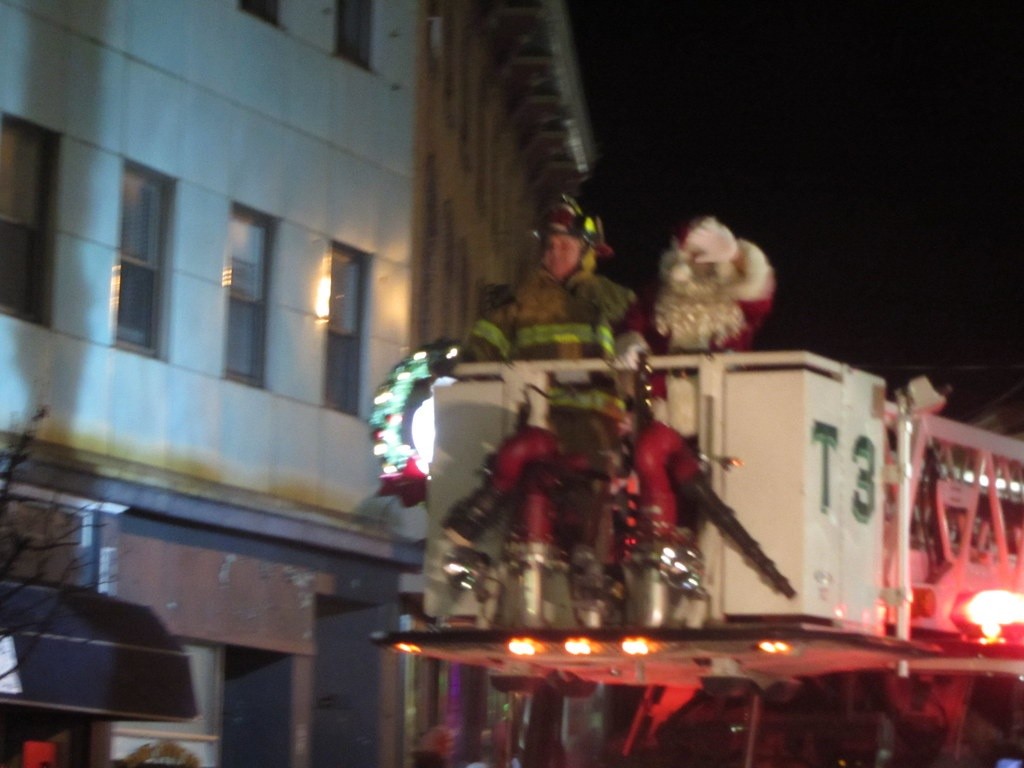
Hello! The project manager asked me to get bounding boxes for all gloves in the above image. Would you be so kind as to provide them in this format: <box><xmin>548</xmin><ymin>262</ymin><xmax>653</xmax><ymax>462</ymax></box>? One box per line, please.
<box><xmin>684</xmin><ymin>217</ymin><xmax>738</xmax><ymax>266</ymax></box>
<box><xmin>443</xmin><ymin>343</ymin><xmax>483</xmax><ymax>370</ymax></box>
<box><xmin>605</xmin><ymin>326</ymin><xmax>649</xmax><ymax>372</ymax></box>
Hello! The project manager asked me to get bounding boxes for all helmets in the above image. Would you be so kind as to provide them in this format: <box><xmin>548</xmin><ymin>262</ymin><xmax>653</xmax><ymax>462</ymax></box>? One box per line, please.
<box><xmin>526</xmin><ymin>194</ymin><xmax>613</xmax><ymax>257</ymax></box>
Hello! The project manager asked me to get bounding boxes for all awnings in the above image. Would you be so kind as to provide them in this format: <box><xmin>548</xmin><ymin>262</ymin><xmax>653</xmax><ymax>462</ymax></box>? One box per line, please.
<box><xmin>0</xmin><ymin>574</ymin><xmax>200</xmax><ymax>724</ymax></box>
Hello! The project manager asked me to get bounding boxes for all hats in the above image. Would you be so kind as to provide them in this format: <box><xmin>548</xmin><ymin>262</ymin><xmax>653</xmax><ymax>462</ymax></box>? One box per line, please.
<box><xmin>671</xmin><ymin>210</ymin><xmax>710</xmax><ymax>247</ymax></box>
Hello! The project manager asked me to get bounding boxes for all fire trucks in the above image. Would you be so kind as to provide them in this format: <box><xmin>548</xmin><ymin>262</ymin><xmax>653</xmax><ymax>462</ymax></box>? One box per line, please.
<box><xmin>371</xmin><ymin>349</ymin><xmax>1024</xmax><ymax>768</ymax></box>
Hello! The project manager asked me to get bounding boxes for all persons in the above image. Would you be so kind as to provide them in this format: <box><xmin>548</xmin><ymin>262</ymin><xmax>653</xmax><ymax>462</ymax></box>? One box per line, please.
<box><xmin>423</xmin><ymin>195</ymin><xmax>699</xmax><ymax>639</ymax></box>
<box><xmin>411</xmin><ymin>725</ymin><xmax>459</xmax><ymax>767</ymax></box>
